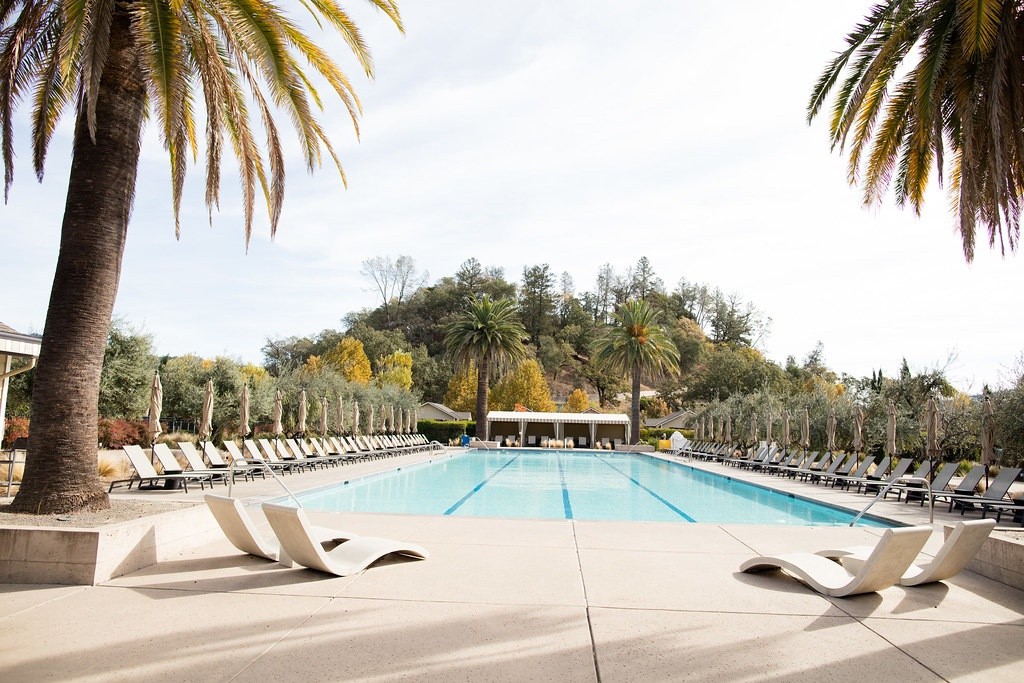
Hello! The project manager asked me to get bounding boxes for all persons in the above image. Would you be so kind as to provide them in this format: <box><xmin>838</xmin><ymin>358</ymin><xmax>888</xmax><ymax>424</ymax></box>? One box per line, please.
<box><xmin>661</xmin><ymin>433</ymin><xmax>668</xmax><ymax>439</ymax></box>
<box><xmin>725</xmin><ymin>415</ymin><xmax>732</xmax><ymax>453</ymax></box>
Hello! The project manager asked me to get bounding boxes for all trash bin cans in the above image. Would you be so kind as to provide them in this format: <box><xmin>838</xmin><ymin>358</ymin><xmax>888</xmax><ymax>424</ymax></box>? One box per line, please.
<box><xmin>462</xmin><ymin>435</ymin><xmax>471</xmax><ymax>447</ymax></box>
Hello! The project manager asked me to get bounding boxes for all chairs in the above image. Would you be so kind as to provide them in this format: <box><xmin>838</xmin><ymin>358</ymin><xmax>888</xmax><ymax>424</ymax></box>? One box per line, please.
<box><xmin>659</xmin><ymin>441</ymin><xmax>1024</xmax><ymax>528</ymax></box>
<box><xmin>108</xmin><ymin>431</ymin><xmax>440</xmax><ymax>492</ymax></box>
<box><xmin>204</xmin><ymin>495</ymin><xmax>357</xmax><ymax>562</ymax></box>
<box><xmin>813</xmin><ymin>519</ymin><xmax>997</xmax><ymax>586</ymax></box>
<box><xmin>495</xmin><ymin>436</ymin><xmax>621</xmax><ymax>449</ymax></box>
<box><xmin>739</xmin><ymin>527</ymin><xmax>933</xmax><ymax>597</ymax></box>
<box><xmin>261</xmin><ymin>503</ymin><xmax>427</xmax><ymax>576</ymax></box>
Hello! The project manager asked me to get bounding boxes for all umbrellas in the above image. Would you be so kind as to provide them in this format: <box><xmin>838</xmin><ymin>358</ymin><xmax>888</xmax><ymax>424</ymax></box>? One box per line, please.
<box><xmin>405</xmin><ymin>409</ymin><xmax>411</xmax><ymax>436</ymax></box>
<box><xmin>822</xmin><ymin>406</ymin><xmax>836</xmax><ymax>481</ymax></box>
<box><xmin>708</xmin><ymin>416</ymin><xmax>714</xmax><ymax>442</ymax></box>
<box><xmin>380</xmin><ymin>403</ymin><xmax>387</xmax><ymax>438</ymax></box>
<box><xmin>294</xmin><ymin>388</ymin><xmax>307</xmax><ymax>466</ymax></box>
<box><xmin>850</xmin><ymin>386</ymin><xmax>999</xmax><ymax>512</ymax></box>
<box><xmin>234</xmin><ymin>382</ymin><xmax>252</xmax><ymax>475</ymax></box>
<box><xmin>270</xmin><ymin>387</ymin><xmax>283</xmax><ymax>470</ymax></box>
<box><xmin>766</xmin><ymin>413</ymin><xmax>773</xmax><ymax>469</ymax></box>
<box><xmin>389</xmin><ymin>404</ymin><xmax>396</xmax><ymax>436</ymax></box>
<box><xmin>138</xmin><ymin>371</ymin><xmax>164</xmax><ymax>490</ymax></box>
<box><xmin>397</xmin><ymin>405</ymin><xmax>403</xmax><ymax>436</ymax></box>
<box><xmin>699</xmin><ymin>416</ymin><xmax>704</xmax><ymax>441</ymax></box>
<box><xmin>317</xmin><ymin>395</ymin><xmax>328</xmax><ymax>464</ymax></box>
<box><xmin>191</xmin><ymin>376</ymin><xmax>214</xmax><ymax>481</ymax></box>
<box><xmin>798</xmin><ymin>408</ymin><xmax>811</xmax><ymax>477</ymax></box>
<box><xmin>750</xmin><ymin>413</ymin><xmax>757</xmax><ymax>458</ymax></box>
<box><xmin>337</xmin><ymin>396</ymin><xmax>343</xmax><ymax>448</ymax></box>
<box><xmin>717</xmin><ymin>416</ymin><xmax>723</xmax><ymax>442</ymax></box>
<box><xmin>412</xmin><ymin>409</ymin><xmax>418</xmax><ymax>434</ymax></box>
<box><xmin>781</xmin><ymin>409</ymin><xmax>791</xmax><ymax>462</ymax></box>
<box><xmin>368</xmin><ymin>403</ymin><xmax>373</xmax><ymax>442</ymax></box>
<box><xmin>353</xmin><ymin>402</ymin><xmax>358</xmax><ymax>442</ymax></box>
<box><xmin>693</xmin><ymin>420</ymin><xmax>699</xmax><ymax>441</ymax></box>
